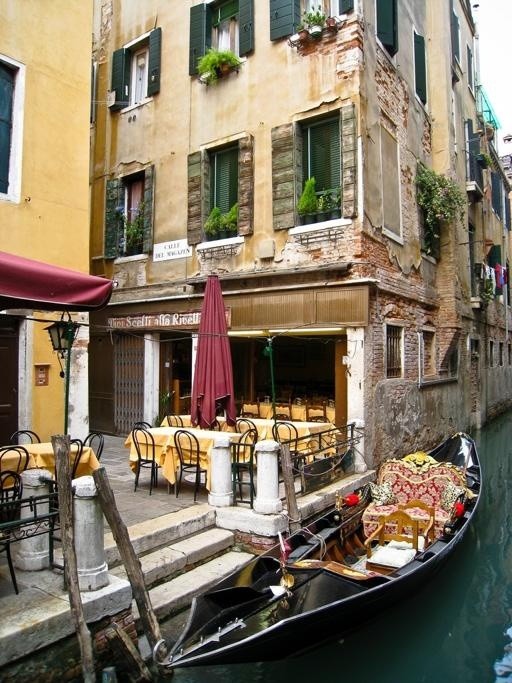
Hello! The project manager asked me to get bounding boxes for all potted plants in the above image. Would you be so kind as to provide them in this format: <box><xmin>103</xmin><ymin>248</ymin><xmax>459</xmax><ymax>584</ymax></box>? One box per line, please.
<box><xmin>204</xmin><ymin>202</ymin><xmax>237</xmax><ymax>241</ymax></box>
<box><xmin>294</xmin><ymin>6</ymin><xmax>336</xmax><ymax>41</ymax></box>
<box><xmin>196</xmin><ymin>46</ymin><xmax>241</xmax><ymax>87</ymax></box>
<box><xmin>296</xmin><ymin>176</ymin><xmax>341</xmax><ymax>225</ymax></box>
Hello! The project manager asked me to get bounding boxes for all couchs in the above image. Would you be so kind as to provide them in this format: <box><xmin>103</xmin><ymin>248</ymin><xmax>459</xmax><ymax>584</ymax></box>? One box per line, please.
<box><xmin>360</xmin><ymin>451</ymin><xmax>468</xmax><ymax>542</ymax></box>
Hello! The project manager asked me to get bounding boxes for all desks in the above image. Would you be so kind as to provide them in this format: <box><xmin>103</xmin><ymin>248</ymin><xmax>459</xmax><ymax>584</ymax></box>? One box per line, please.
<box><xmin>124</xmin><ymin>395</ymin><xmax>342</xmax><ymax>502</ymax></box>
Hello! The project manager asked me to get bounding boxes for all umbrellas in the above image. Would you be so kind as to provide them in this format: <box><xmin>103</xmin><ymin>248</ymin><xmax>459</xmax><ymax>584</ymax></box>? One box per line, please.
<box><xmin>185</xmin><ymin>272</ymin><xmax>239</xmax><ymax>432</ymax></box>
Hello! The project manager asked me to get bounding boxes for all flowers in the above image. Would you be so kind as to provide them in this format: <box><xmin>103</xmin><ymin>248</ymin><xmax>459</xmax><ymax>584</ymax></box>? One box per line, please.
<box><xmin>115</xmin><ymin>202</ymin><xmax>146</xmax><ymax>248</ymax></box>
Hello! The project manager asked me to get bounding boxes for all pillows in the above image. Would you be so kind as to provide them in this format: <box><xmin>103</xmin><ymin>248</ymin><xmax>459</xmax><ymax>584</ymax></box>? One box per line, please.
<box><xmin>436</xmin><ymin>480</ymin><xmax>467</xmax><ymax>515</ymax></box>
<box><xmin>369</xmin><ymin>480</ymin><xmax>399</xmax><ymax>506</ymax></box>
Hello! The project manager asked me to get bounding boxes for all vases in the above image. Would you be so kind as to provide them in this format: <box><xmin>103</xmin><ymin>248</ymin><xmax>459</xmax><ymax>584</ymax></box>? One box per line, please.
<box><xmin>127</xmin><ymin>248</ymin><xmax>141</xmax><ymax>255</ymax></box>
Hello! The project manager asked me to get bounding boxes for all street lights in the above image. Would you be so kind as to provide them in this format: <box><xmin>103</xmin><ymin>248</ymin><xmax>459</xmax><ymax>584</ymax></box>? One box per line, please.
<box><xmin>42</xmin><ymin>311</ymin><xmax>81</xmax><ymax>435</ymax></box>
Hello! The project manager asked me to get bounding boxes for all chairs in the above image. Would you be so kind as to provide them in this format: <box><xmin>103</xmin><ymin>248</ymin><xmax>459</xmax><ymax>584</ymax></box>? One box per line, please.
<box><xmin>364</xmin><ymin>510</ymin><xmax>418</xmax><ymax>575</ymax></box>
<box><xmin>386</xmin><ymin>499</ymin><xmax>435</xmax><ymax>553</ymax></box>
<box><xmin>0</xmin><ymin>430</ymin><xmax>104</xmax><ymax>594</ymax></box>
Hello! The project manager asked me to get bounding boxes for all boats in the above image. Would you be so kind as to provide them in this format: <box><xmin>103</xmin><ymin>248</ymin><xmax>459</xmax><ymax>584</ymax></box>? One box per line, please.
<box><xmin>152</xmin><ymin>431</ymin><xmax>483</xmax><ymax>670</ymax></box>
